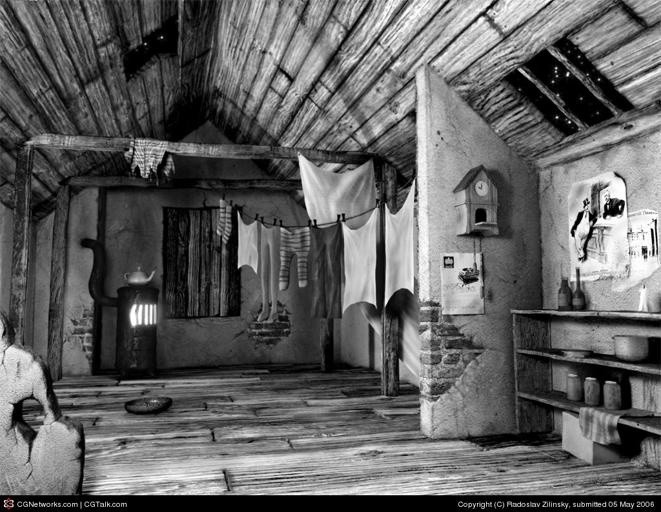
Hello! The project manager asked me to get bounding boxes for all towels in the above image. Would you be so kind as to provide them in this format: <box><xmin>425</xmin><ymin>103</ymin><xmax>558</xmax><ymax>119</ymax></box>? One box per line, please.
<box><xmin>331</xmin><ymin>179</ymin><xmax>418</xmax><ymax>313</ymax></box>
<box><xmin>236</xmin><ymin>211</ymin><xmax>259</xmax><ymax>274</ymax></box>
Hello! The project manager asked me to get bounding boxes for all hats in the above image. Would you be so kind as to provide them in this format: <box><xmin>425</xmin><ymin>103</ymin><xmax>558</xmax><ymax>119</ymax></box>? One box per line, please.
<box><xmin>582</xmin><ymin>198</ymin><xmax>590</xmax><ymax>208</ymax></box>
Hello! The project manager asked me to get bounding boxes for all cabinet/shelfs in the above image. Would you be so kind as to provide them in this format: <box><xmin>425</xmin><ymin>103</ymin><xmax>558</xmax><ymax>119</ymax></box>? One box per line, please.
<box><xmin>507</xmin><ymin>309</ymin><xmax>660</xmax><ymax>434</ymax></box>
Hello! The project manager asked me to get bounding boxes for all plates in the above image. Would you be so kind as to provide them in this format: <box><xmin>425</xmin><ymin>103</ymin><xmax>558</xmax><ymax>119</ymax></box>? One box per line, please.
<box><xmin>563</xmin><ymin>349</ymin><xmax>592</xmax><ymax>358</ymax></box>
<box><xmin>125</xmin><ymin>397</ymin><xmax>171</xmax><ymax>414</ymax></box>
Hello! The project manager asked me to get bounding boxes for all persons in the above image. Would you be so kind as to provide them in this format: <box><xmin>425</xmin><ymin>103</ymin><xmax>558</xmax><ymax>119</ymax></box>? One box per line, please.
<box><xmin>602</xmin><ymin>191</ymin><xmax>625</xmax><ymax>220</ymax></box>
<box><xmin>571</xmin><ymin>199</ymin><xmax>597</xmax><ymax>262</ymax></box>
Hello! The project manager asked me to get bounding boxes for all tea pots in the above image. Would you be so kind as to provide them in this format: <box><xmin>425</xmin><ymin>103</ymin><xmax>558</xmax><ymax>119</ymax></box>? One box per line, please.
<box><xmin>123</xmin><ymin>266</ymin><xmax>155</xmax><ymax>286</ymax></box>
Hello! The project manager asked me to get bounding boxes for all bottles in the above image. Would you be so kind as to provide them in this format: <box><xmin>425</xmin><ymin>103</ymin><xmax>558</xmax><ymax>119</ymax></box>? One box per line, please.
<box><xmin>564</xmin><ymin>374</ymin><xmax>622</xmax><ymax>411</ymax></box>
<box><xmin>571</xmin><ymin>267</ymin><xmax>586</xmax><ymax>310</ymax></box>
<box><xmin>557</xmin><ymin>277</ymin><xmax>573</xmax><ymax>311</ymax></box>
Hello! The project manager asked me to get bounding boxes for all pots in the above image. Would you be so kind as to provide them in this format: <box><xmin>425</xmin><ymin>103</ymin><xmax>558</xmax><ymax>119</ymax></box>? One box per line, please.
<box><xmin>613</xmin><ymin>336</ymin><xmax>649</xmax><ymax>361</ymax></box>
